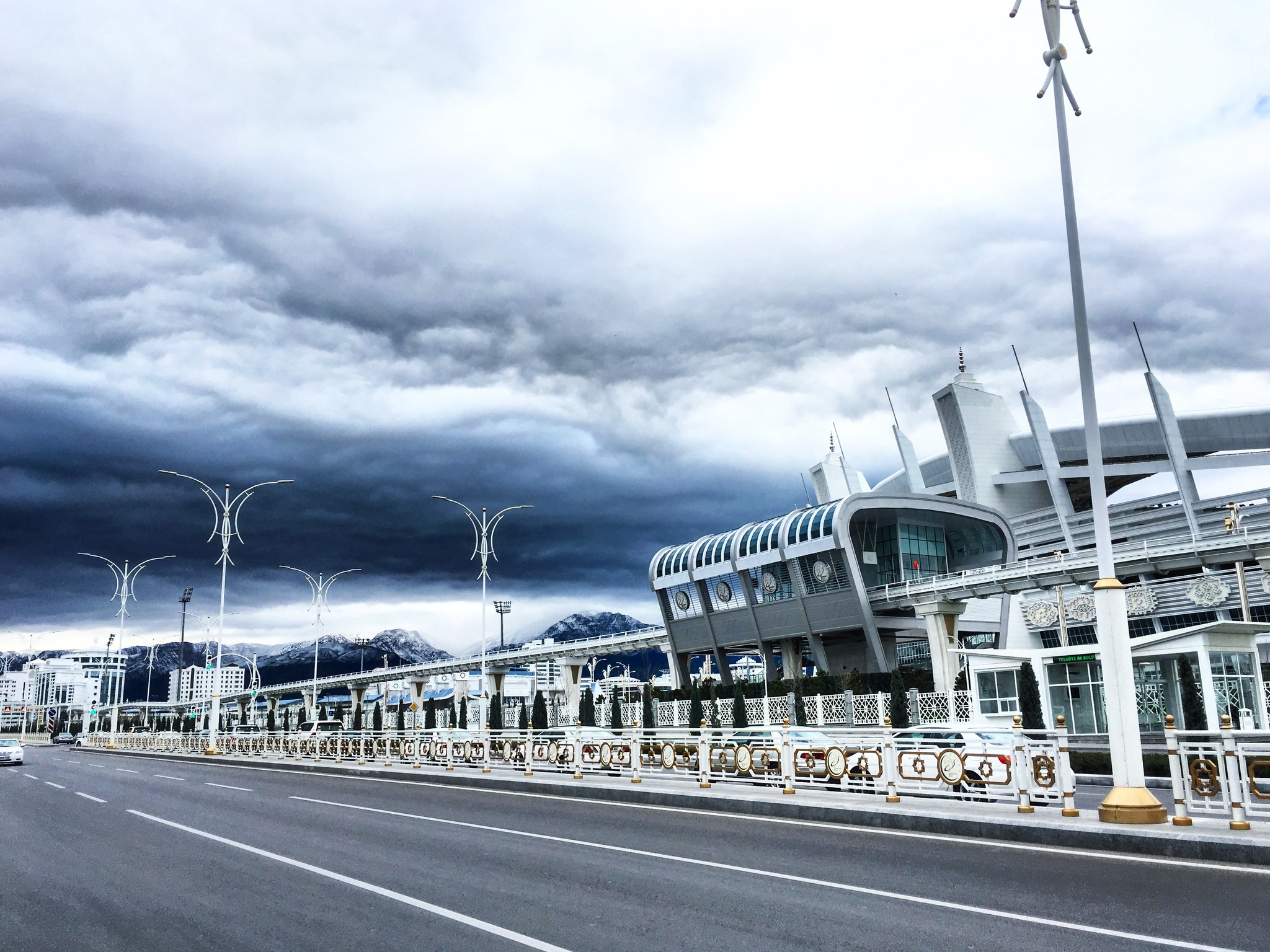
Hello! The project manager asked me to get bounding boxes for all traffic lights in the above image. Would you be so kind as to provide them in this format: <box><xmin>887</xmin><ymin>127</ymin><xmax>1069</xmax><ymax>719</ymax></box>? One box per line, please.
<box><xmin>90</xmin><ymin>699</ymin><xmax>97</xmax><ymax>715</ymax></box>
<box><xmin>252</xmin><ymin>681</ymin><xmax>257</xmax><ymax>696</ymax></box>
<box><xmin>190</xmin><ymin>705</ymin><xmax>195</xmax><ymax>718</ymax></box>
<box><xmin>207</xmin><ymin>655</ymin><xmax>212</xmax><ymax>669</ymax></box>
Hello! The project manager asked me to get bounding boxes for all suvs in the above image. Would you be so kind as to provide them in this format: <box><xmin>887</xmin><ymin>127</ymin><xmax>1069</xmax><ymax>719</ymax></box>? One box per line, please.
<box><xmin>219</xmin><ymin>724</ymin><xmax>262</xmax><ymax>744</ymax></box>
<box><xmin>125</xmin><ymin>726</ymin><xmax>152</xmax><ymax>739</ymax></box>
<box><xmin>287</xmin><ymin>719</ymin><xmax>346</xmax><ymax>751</ymax></box>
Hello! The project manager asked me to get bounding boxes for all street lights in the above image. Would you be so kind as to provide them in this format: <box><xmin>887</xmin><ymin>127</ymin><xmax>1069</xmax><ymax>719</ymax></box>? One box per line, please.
<box><xmin>2</xmin><ymin>630</ymin><xmax>61</xmax><ymax>740</ymax></box>
<box><xmin>433</xmin><ymin>495</ymin><xmax>536</xmax><ymax>741</ymax></box>
<box><xmin>76</xmin><ymin>552</ymin><xmax>178</xmax><ymax>751</ymax></box>
<box><xmin>101</xmin><ymin>633</ymin><xmax>115</xmax><ymax>706</ymax></box>
<box><xmin>178</xmin><ymin>611</ymin><xmax>240</xmax><ymax>753</ymax></box>
<box><xmin>176</xmin><ymin>586</ymin><xmax>194</xmax><ymax>701</ymax></box>
<box><xmin>129</xmin><ymin>633</ymin><xmax>180</xmax><ymax>727</ymax></box>
<box><xmin>381</xmin><ymin>656</ymin><xmax>389</xmax><ymax>713</ymax></box>
<box><xmin>493</xmin><ymin>601</ymin><xmax>512</xmax><ymax>648</ymax></box>
<box><xmin>158</xmin><ymin>468</ymin><xmax>296</xmax><ymax>753</ymax></box>
<box><xmin>270</xmin><ymin>697</ymin><xmax>279</xmax><ymax>727</ymax></box>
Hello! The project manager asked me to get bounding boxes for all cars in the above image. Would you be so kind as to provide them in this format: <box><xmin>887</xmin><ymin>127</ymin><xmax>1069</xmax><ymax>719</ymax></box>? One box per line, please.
<box><xmin>75</xmin><ymin>732</ymin><xmax>111</xmax><ymax>747</ymax></box>
<box><xmin>73</xmin><ymin>732</ymin><xmax>87</xmax><ymax>742</ymax></box>
<box><xmin>0</xmin><ymin>738</ymin><xmax>26</xmax><ymax>766</ymax></box>
<box><xmin>695</xmin><ymin>724</ymin><xmax>840</xmax><ymax>788</ymax></box>
<box><xmin>845</xmin><ymin>725</ymin><xmax>1077</xmax><ymax>806</ymax></box>
<box><xmin>186</xmin><ymin>729</ymin><xmax>221</xmax><ymax>737</ymax></box>
<box><xmin>325</xmin><ymin>730</ymin><xmax>376</xmax><ymax>762</ymax></box>
<box><xmin>400</xmin><ymin>727</ymin><xmax>480</xmax><ymax>768</ymax></box>
<box><xmin>157</xmin><ymin>731</ymin><xmax>185</xmax><ymax>739</ymax></box>
<box><xmin>510</xmin><ymin>724</ymin><xmax>623</xmax><ymax>776</ymax></box>
<box><xmin>53</xmin><ymin>732</ymin><xmax>73</xmax><ymax>745</ymax></box>
